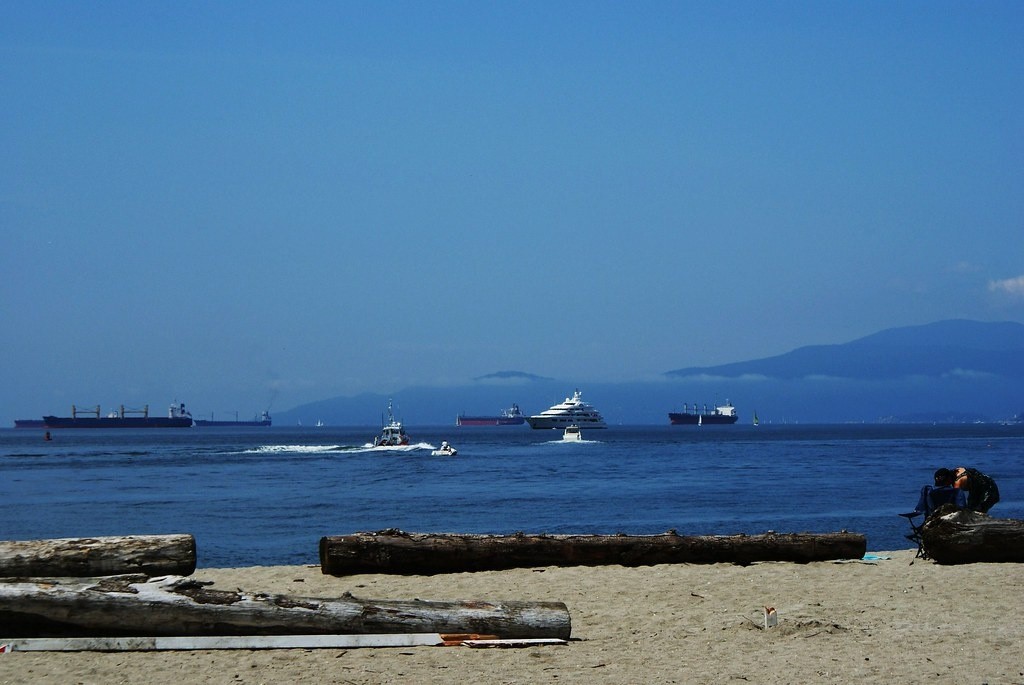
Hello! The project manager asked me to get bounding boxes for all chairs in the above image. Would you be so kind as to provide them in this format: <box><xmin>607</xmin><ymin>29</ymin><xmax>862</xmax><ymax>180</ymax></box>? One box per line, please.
<box><xmin>898</xmin><ymin>481</ymin><xmax>965</xmax><ymax>566</ymax></box>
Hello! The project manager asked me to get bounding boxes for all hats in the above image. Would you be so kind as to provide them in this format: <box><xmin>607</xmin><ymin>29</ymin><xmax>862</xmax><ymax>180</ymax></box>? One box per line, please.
<box><xmin>934</xmin><ymin>468</ymin><xmax>950</xmax><ymax>486</ymax></box>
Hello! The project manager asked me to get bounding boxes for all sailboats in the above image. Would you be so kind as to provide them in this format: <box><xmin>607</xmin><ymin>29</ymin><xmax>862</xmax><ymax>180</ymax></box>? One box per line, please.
<box><xmin>296</xmin><ymin>416</ymin><xmax>304</xmax><ymax>427</ymax></box>
<box><xmin>315</xmin><ymin>419</ymin><xmax>324</xmax><ymax>427</ymax></box>
<box><xmin>752</xmin><ymin>410</ymin><xmax>760</xmax><ymax>432</ymax></box>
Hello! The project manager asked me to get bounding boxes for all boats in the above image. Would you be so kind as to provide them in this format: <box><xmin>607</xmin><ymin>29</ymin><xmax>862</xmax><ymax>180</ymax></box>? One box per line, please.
<box><xmin>562</xmin><ymin>424</ymin><xmax>582</xmax><ymax>441</ymax></box>
<box><xmin>195</xmin><ymin>419</ymin><xmax>272</xmax><ymax>427</ymax></box>
<box><xmin>374</xmin><ymin>422</ymin><xmax>411</xmax><ymax>446</ymax></box>
<box><xmin>456</xmin><ymin>403</ymin><xmax>525</xmax><ymax>426</ymax></box>
<box><xmin>431</xmin><ymin>445</ymin><xmax>457</xmax><ymax>457</ymax></box>
<box><xmin>42</xmin><ymin>404</ymin><xmax>194</xmax><ymax>428</ymax></box>
<box><xmin>14</xmin><ymin>419</ymin><xmax>47</xmax><ymax>428</ymax></box>
<box><xmin>666</xmin><ymin>391</ymin><xmax>739</xmax><ymax>424</ymax></box>
<box><xmin>525</xmin><ymin>388</ymin><xmax>609</xmax><ymax>428</ymax></box>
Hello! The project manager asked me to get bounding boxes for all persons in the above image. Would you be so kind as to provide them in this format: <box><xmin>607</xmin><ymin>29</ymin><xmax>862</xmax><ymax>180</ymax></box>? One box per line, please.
<box><xmin>935</xmin><ymin>467</ymin><xmax>999</xmax><ymax>515</ymax></box>
<box><xmin>440</xmin><ymin>439</ymin><xmax>451</xmax><ymax>452</ymax></box>
